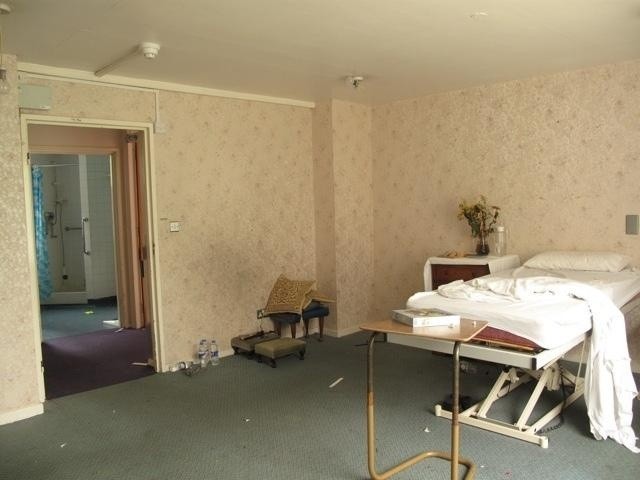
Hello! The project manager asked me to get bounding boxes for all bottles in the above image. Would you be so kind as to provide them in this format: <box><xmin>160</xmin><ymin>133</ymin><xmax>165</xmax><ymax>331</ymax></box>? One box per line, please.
<box><xmin>169</xmin><ymin>360</ymin><xmax>195</xmax><ymax>372</ymax></box>
<box><xmin>200</xmin><ymin>339</ymin><xmax>219</xmax><ymax>368</ymax></box>
<box><xmin>495</xmin><ymin>226</ymin><xmax>507</xmax><ymax>256</ymax></box>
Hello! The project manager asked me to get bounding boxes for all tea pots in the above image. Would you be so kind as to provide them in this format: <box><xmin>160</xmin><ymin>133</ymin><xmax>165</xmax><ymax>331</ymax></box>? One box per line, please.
<box><xmin>473</xmin><ymin>236</ymin><xmax>490</xmax><ymax>255</ymax></box>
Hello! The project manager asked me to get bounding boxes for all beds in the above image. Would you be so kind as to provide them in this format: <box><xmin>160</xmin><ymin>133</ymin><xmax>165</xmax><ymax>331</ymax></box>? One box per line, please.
<box><xmin>388</xmin><ymin>252</ymin><xmax>640</xmax><ymax>448</ymax></box>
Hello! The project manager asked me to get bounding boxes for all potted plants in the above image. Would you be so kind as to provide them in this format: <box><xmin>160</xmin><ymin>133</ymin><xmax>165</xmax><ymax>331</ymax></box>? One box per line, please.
<box><xmin>457</xmin><ymin>195</ymin><xmax>500</xmax><ymax>255</ymax></box>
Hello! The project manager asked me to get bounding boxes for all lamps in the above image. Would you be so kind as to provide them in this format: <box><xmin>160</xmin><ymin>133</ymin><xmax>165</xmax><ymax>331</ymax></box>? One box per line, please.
<box><xmin>0</xmin><ymin>67</ymin><xmax>11</xmax><ymax>95</ymax></box>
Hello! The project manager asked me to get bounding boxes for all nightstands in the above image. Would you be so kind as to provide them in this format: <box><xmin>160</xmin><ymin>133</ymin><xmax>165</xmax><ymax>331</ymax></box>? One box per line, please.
<box><xmin>424</xmin><ymin>255</ymin><xmax>520</xmax><ymax>290</ymax></box>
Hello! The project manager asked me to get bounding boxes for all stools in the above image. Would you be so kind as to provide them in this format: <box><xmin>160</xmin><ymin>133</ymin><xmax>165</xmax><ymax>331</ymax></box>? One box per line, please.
<box><xmin>271</xmin><ymin>306</ymin><xmax>329</xmax><ymax>342</ymax></box>
<box><xmin>254</xmin><ymin>337</ymin><xmax>307</xmax><ymax>368</ymax></box>
<box><xmin>231</xmin><ymin>332</ymin><xmax>280</xmax><ymax>360</ymax></box>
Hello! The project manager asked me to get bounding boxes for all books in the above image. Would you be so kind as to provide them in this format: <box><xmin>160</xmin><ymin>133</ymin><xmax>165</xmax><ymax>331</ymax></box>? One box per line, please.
<box><xmin>392</xmin><ymin>308</ymin><xmax>460</xmax><ymax>328</ymax></box>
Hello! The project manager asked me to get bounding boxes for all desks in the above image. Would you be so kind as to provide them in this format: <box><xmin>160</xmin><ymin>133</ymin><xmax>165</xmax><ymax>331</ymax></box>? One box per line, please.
<box><xmin>358</xmin><ymin>318</ymin><xmax>488</xmax><ymax>480</ymax></box>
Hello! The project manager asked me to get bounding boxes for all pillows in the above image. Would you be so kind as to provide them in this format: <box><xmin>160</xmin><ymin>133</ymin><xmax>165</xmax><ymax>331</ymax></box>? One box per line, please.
<box><xmin>263</xmin><ymin>273</ymin><xmax>316</xmax><ymax>317</ymax></box>
<box><xmin>303</xmin><ymin>290</ymin><xmax>337</xmax><ymax>311</ymax></box>
<box><xmin>521</xmin><ymin>252</ymin><xmax>631</xmax><ymax>272</ymax></box>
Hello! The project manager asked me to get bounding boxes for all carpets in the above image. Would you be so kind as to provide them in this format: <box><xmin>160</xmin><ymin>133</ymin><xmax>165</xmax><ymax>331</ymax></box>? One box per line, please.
<box><xmin>42</xmin><ymin>328</ymin><xmax>154</xmax><ymax>401</ymax></box>
